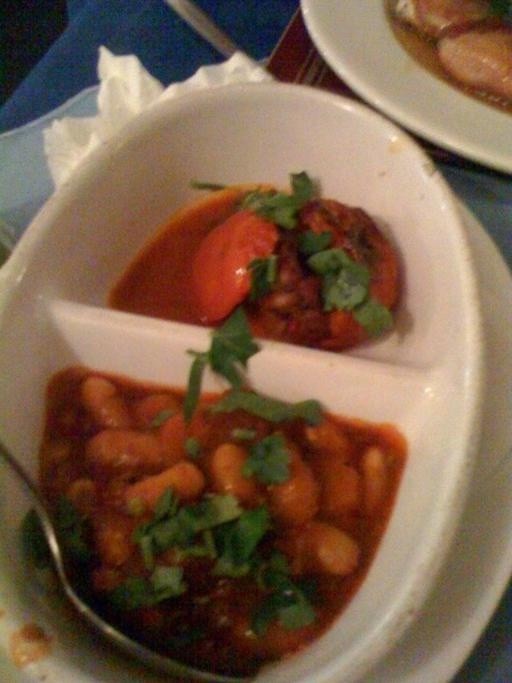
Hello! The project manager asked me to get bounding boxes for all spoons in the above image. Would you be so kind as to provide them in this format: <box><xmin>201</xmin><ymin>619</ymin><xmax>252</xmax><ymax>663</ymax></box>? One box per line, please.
<box><xmin>3</xmin><ymin>442</ymin><xmax>250</xmax><ymax>678</ymax></box>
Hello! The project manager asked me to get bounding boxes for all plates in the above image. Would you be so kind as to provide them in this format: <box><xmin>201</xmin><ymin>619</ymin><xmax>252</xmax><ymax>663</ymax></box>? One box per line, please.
<box><xmin>362</xmin><ymin>173</ymin><xmax>512</xmax><ymax>681</ymax></box>
<box><xmin>2</xmin><ymin>75</ymin><xmax>485</xmax><ymax>683</ymax></box>
<box><xmin>294</xmin><ymin>0</ymin><xmax>512</xmax><ymax>176</ymax></box>
